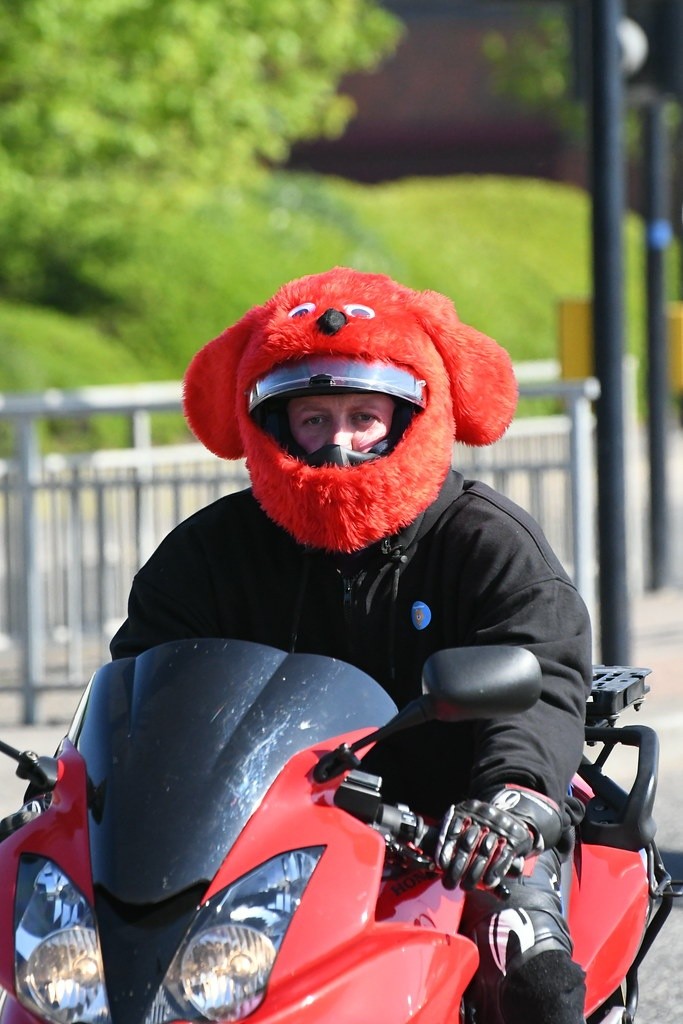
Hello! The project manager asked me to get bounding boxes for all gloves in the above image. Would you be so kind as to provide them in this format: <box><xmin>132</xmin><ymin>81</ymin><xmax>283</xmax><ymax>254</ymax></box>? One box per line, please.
<box><xmin>433</xmin><ymin>790</ymin><xmax>566</xmax><ymax>892</ymax></box>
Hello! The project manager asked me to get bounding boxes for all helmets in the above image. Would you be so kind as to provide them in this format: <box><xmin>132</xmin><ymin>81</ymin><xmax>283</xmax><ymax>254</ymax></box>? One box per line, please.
<box><xmin>248</xmin><ymin>353</ymin><xmax>427</xmax><ymax>467</ymax></box>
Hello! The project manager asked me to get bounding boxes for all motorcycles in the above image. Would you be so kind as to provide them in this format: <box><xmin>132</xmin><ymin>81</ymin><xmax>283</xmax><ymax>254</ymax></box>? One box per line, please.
<box><xmin>0</xmin><ymin>643</ymin><xmax>683</xmax><ymax>1024</ymax></box>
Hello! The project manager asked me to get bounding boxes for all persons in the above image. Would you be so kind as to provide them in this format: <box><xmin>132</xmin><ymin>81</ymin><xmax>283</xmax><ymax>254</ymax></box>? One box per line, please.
<box><xmin>105</xmin><ymin>262</ymin><xmax>593</xmax><ymax>1024</ymax></box>
